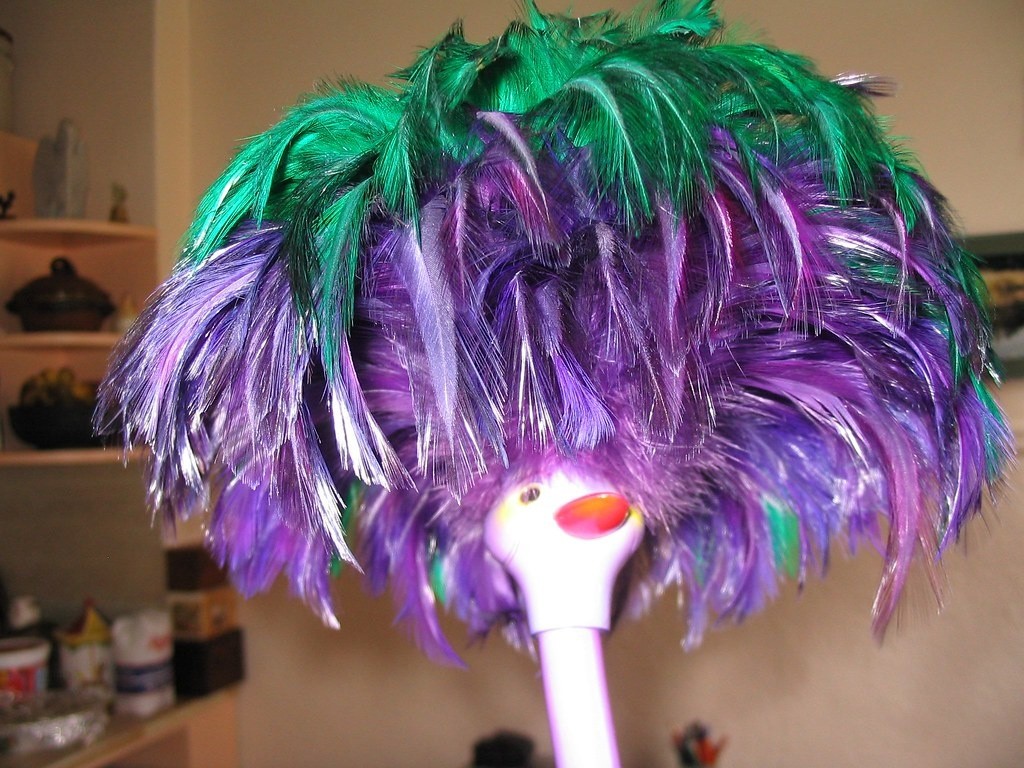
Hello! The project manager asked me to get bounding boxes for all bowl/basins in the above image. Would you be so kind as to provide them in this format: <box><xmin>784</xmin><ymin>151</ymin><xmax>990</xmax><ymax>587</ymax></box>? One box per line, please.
<box><xmin>9</xmin><ymin>405</ymin><xmax>138</xmax><ymax>448</ymax></box>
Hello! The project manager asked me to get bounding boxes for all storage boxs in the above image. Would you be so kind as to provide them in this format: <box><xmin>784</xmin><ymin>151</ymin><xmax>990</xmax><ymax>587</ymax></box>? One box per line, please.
<box><xmin>165</xmin><ymin>540</ymin><xmax>245</xmax><ymax>702</ymax></box>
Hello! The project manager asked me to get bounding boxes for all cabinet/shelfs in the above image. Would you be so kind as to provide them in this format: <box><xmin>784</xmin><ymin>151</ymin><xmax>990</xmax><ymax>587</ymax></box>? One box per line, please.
<box><xmin>0</xmin><ymin>129</ymin><xmax>159</xmax><ymax>463</ymax></box>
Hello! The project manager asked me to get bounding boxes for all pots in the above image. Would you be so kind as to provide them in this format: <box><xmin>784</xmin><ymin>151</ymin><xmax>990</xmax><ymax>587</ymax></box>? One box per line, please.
<box><xmin>4</xmin><ymin>258</ymin><xmax>116</xmax><ymax>331</ymax></box>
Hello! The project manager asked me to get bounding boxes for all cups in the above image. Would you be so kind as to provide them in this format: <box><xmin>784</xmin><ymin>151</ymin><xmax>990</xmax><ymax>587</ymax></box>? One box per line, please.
<box><xmin>0</xmin><ymin>638</ymin><xmax>49</xmax><ymax>717</ymax></box>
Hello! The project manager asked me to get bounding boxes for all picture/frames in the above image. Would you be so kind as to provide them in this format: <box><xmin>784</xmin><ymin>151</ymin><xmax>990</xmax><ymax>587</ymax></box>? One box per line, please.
<box><xmin>960</xmin><ymin>233</ymin><xmax>1023</xmax><ymax>381</ymax></box>
